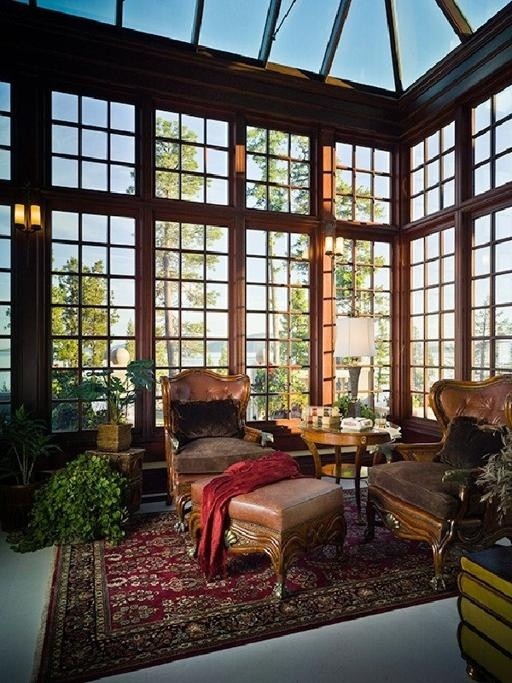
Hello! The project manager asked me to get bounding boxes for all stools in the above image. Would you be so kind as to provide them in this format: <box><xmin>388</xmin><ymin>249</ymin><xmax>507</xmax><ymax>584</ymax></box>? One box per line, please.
<box><xmin>187</xmin><ymin>468</ymin><xmax>349</xmax><ymax>603</ymax></box>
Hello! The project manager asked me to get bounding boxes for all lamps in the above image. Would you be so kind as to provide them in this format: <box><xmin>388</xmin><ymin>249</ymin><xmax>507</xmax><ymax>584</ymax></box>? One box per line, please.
<box><xmin>321</xmin><ymin>315</ymin><xmax>377</xmax><ymax>478</ymax></box>
<box><xmin>12</xmin><ymin>203</ymin><xmax>43</xmax><ymax>241</ymax></box>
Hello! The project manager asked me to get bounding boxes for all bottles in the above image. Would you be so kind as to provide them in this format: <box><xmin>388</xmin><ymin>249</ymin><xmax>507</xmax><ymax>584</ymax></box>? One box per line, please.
<box><xmin>345</xmin><ymin>391</ymin><xmax>351</xmax><ymax>412</ymax></box>
<box><xmin>311</xmin><ymin>407</ymin><xmax>329</xmax><ymax>429</ymax></box>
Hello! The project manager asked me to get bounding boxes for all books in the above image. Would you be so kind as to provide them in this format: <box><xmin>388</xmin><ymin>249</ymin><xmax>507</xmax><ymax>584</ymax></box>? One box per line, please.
<box><xmin>456</xmin><ymin>547</ymin><xmax>511</xmax><ymax>683</ymax></box>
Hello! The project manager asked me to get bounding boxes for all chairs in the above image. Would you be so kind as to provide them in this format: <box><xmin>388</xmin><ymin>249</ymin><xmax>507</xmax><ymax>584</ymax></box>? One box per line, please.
<box><xmin>364</xmin><ymin>373</ymin><xmax>512</xmax><ymax>591</ymax></box>
<box><xmin>160</xmin><ymin>369</ymin><xmax>278</xmax><ymax>531</ymax></box>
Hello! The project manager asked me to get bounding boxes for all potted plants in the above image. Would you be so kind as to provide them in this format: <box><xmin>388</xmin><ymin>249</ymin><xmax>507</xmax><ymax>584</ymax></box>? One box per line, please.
<box><xmin>0</xmin><ymin>403</ymin><xmax>59</xmax><ymax>522</ymax></box>
<box><xmin>72</xmin><ymin>359</ymin><xmax>155</xmax><ymax>454</ymax></box>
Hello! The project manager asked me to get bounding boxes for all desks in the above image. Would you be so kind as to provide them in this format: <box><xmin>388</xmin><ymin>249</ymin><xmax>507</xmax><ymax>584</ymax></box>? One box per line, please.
<box><xmin>86</xmin><ymin>447</ymin><xmax>145</xmax><ymax>514</ymax></box>
<box><xmin>301</xmin><ymin>425</ymin><xmax>392</xmax><ymax>506</ymax></box>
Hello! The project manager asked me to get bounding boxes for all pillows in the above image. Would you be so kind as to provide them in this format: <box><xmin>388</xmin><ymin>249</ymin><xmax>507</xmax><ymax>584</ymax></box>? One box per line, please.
<box><xmin>440</xmin><ymin>418</ymin><xmax>508</xmax><ymax>471</ymax></box>
<box><xmin>170</xmin><ymin>399</ymin><xmax>245</xmax><ymax>449</ymax></box>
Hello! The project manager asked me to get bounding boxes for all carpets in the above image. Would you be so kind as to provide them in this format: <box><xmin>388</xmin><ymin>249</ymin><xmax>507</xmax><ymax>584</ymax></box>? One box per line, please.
<box><xmin>20</xmin><ymin>485</ymin><xmax>463</xmax><ymax>682</ymax></box>
